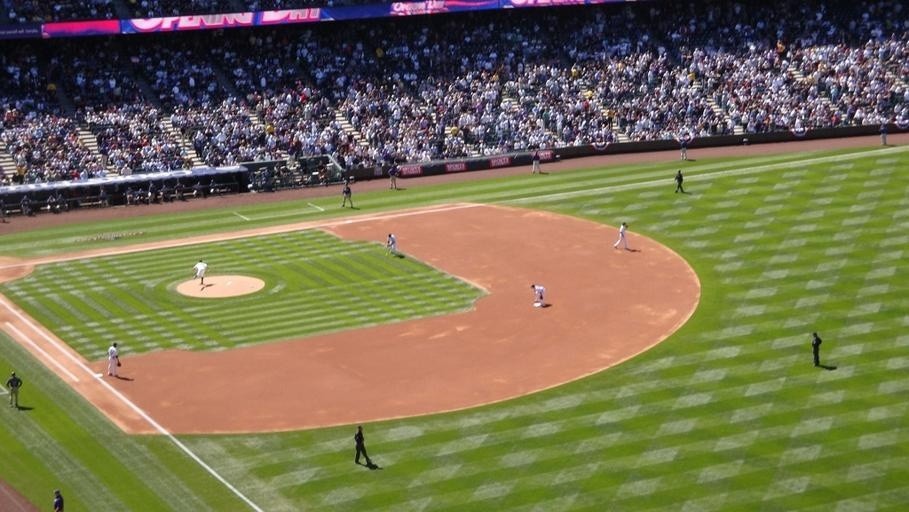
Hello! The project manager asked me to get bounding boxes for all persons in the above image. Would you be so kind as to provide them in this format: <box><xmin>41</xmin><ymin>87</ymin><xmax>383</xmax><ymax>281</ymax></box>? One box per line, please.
<box><xmin>6</xmin><ymin>370</ymin><xmax>23</xmax><ymax>407</ymax></box>
<box><xmin>2</xmin><ymin>1</ymin><xmax>909</xmax><ymax>198</ymax></box>
<box><xmin>108</xmin><ymin>343</ymin><xmax>120</xmax><ymax>376</ymax></box>
<box><xmin>354</xmin><ymin>425</ymin><xmax>372</xmax><ymax>465</ymax></box>
<box><xmin>19</xmin><ymin>195</ymin><xmax>32</xmax><ymax>216</ymax></box>
<box><xmin>193</xmin><ymin>260</ymin><xmax>208</xmax><ymax>283</ymax></box>
<box><xmin>56</xmin><ymin>194</ymin><xmax>66</xmax><ymax>210</ymax></box>
<box><xmin>47</xmin><ymin>195</ymin><xmax>56</xmax><ymax>208</ymax></box>
<box><xmin>614</xmin><ymin>222</ymin><xmax>628</xmax><ymax>248</ymax></box>
<box><xmin>530</xmin><ymin>285</ymin><xmax>546</xmax><ymax>306</ymax></box>
<box><xmin>99</xmin><ymin>189</ymin><xmax>108</xmax><ymax>208</ymax></box>
<box><xmin>126</xmin><ymin>185</ymin><xmax>133</xmax><ymax>205</ymax></box>
<box><xmin>54</xmin><ymin>490</ymin><xmax>64</xmax><ymax>511</ymax></box>
<box><xmin>811</xmin><ymin>333</ymin><xmax>823</xmax><ymax>366</ymax></box>
<box><xmin>341</xmin><ymin>182</ymin><xmax>354</xmax><ymax>208</ymax></box>
<box><xmin>387</xmin><ymin>233</ymin><xmax>398</xmax><ymax>256</ymax></box>
<box><xmin>0</xmin><ymin>198</ymin><xmax>8</xmax><ymax>223</ymax></box>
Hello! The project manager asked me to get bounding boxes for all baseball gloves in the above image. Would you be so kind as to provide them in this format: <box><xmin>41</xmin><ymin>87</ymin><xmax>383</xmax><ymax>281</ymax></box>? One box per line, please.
<box><xmin>117</xmin><ymin>363</ymin><xmax>122</xmax><ymax>367</ymax></box>
